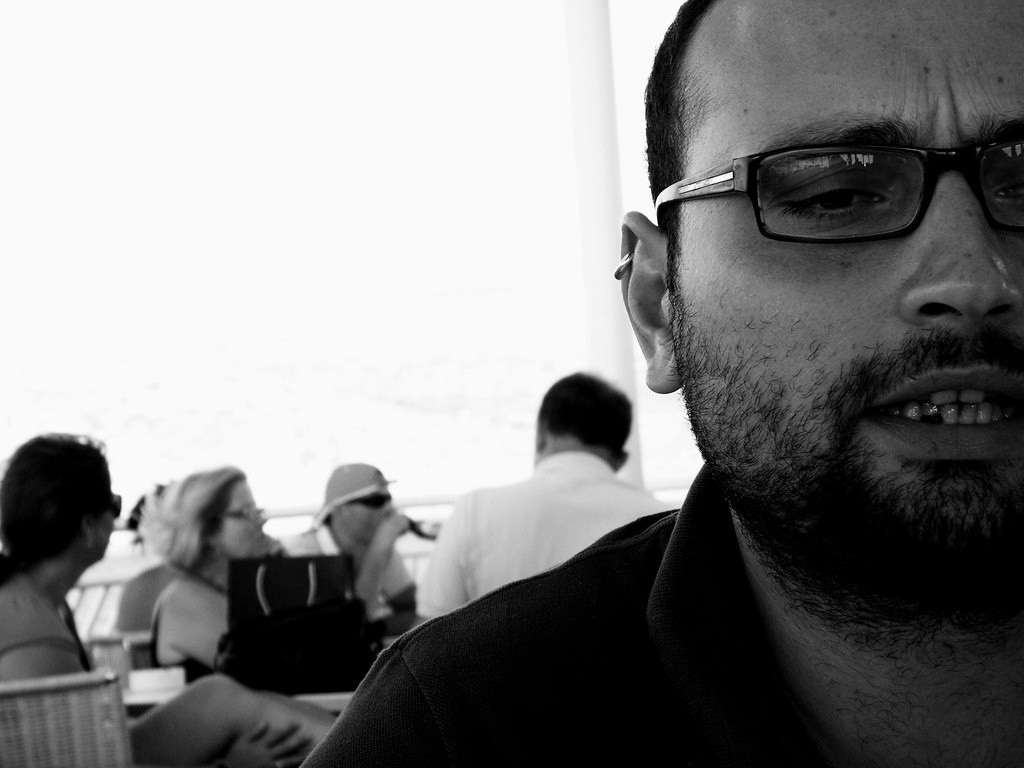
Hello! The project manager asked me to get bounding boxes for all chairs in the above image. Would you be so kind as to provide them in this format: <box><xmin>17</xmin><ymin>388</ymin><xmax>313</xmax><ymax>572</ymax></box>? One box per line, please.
<box><xmin>0</xmin><ymin>665</ymin><xmax>135</xmax><ymax>768</ymax></box>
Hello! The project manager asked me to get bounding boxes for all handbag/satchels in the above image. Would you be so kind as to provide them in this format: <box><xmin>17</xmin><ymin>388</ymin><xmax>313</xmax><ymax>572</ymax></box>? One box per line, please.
<box><xmin>228</xmin><ymin>555</ymin><xmax>350</xmax><ymax>636</ymax></box>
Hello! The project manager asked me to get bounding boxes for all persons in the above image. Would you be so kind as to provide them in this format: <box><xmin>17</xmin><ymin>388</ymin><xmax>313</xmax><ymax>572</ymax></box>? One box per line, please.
<box><xmin>414</xmin><ymin>372</ymin><xmax>674</xmax><ymax>630</ymax></box>
<box><xmin>126</xmin><ymin>460</ymin><xmax>418</xmax><ymax>695</ymax></box>
<box><xmin>294</xmin><ymin>0</ymin><xmax>1023</xmax><ymax>767</ymax></box>
<box><xmin>0</xmin><ymin>433</ymin><xmax>333</xmax><ymax>768</ymax></box>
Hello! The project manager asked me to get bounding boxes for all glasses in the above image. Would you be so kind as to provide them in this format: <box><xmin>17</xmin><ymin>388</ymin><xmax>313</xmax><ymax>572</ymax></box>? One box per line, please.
<box><xmin>347</xmin><ymin>492</ymin><xmax>392</xmax><ymax>510</ymax></box>
<box><xmin>98</xmin><ymin>493</ymin><xmax>122</xmax><ymax>519</ymax></box>
<box><xmin>656</xmin><ymin>134</ymin><xmax>1024</xmax><ymax>243</ymax></box>
<box><xmin>215</xmin><ymin>508</ymin><xmax>263</xmax><ymax>521</ymax></box>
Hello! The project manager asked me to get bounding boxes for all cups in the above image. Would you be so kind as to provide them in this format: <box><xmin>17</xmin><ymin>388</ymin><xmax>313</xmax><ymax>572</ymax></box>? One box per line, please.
<box><xmin>128</xmin><ymin>667</ymin><xmax>188</xmax><ymax>694</ymax></box>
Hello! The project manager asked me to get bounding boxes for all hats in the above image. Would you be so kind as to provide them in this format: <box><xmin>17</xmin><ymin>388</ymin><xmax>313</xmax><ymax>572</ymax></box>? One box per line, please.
<box><xmin>314</xmin><ymin>463</ymin><xmax>388</xmax><ymax>528</ymax></box>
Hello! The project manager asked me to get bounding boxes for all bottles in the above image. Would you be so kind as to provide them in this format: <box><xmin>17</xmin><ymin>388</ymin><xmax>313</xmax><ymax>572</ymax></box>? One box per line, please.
<box><xmin>409</xmin><ymin>520</ymin><xmax>441</xmax><ymax>539</ymax></box>
